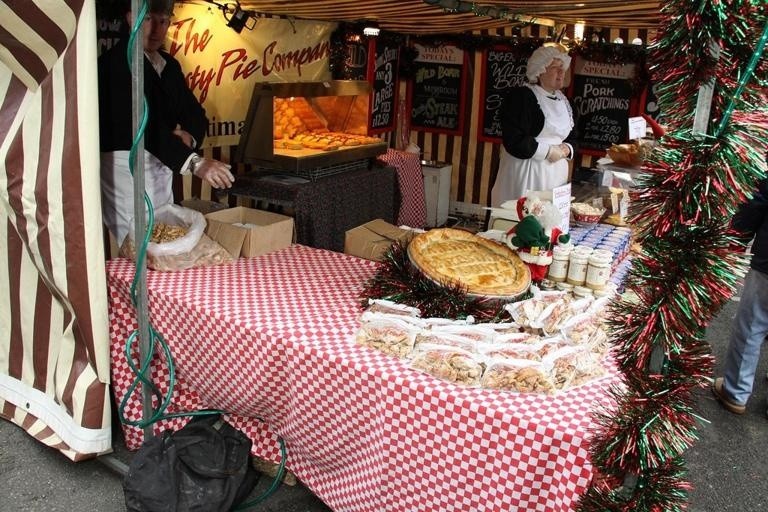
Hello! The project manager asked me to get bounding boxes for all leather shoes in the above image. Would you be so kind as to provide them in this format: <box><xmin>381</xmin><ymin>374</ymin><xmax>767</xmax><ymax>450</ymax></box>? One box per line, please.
<box><xmin>711</xmin><ymin>375</ymin><xmax>746</xmax><ymax>415</ymax></box>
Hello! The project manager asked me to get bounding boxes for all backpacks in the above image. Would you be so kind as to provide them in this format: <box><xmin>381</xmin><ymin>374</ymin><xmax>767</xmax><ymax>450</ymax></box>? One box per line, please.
<box><xmin>122</xmin><ymin>409</ymin><xmax>266</xmax><ymax>512</ymax></box>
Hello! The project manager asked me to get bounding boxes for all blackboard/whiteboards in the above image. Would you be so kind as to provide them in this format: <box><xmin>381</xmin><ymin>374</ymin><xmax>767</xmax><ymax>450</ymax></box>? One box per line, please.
<box><xmin>478</xmin><ymin>36</ymin><xmax>565</xmax><ymax>143</ymax></box>
<box><xmin>406</xmin><ymin>41</ymin><xmax>469</xmax><ymax>136</ymax></box>
<box><xmin>368</xmin><ymin>35</ymin><xmax>401</xmax><ymax>134</ymax></box>
<box><xmin>567</xmin><ymin>40</ymin><xmax>650</xmax><ymax>157</ymax></box>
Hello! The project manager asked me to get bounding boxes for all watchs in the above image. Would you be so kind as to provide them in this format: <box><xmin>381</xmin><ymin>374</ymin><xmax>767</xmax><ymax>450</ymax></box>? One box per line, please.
<box><xmin>189</xmin><ymin>155</ymin><xmax>200</xmax><ymax>175</ymax></box>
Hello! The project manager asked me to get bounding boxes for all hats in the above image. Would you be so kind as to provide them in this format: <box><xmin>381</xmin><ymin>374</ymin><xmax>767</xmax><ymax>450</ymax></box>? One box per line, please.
<box><xmin>522</xmin><ymin>43</ymin><xmax>575</xmax><ymax>87</ymax></box>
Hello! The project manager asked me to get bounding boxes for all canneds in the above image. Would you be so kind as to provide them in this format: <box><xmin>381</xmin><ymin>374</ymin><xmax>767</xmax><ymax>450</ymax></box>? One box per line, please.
<box><xmin>540</xmin><ymin>222</ymin><xmax>632</xmax><ymax>300</ymax></box>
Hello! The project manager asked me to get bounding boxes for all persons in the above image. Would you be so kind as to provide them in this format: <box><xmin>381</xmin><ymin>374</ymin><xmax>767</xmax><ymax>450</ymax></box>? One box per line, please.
<box><xmin>487</xmin><ymin>46</ymin><xmax>585</xmax><ymax>236</ymax></box>
<box><xmin>712</xmin><ymin>179</ymin><xmax>768</xmax><ymax>414</ymax></box>
<box><xmin>97</xmin><ymin>0</ymin><xmax>236</xmax><ymax>249</ymax></box>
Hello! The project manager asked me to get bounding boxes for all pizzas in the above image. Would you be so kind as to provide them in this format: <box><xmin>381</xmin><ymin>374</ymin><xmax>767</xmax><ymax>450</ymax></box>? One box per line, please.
<box><xmin>408</xmin><ymin>227</ymin><xmax>529</xmax><ymax>296</ymax></box>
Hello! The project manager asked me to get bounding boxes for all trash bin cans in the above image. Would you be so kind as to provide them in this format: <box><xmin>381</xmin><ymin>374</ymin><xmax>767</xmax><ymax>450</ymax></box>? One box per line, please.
<box><xmin>419</xmin><ymin>160</ymin><xmax>453</xmax><ymax>227</ymax></box>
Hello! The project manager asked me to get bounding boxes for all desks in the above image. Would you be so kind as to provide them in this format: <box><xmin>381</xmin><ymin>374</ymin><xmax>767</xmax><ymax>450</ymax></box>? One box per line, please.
<box><xmin>225</xmin><ymin>161</ymin><xmax>400</xmax><ymax>254</ymax></box>
<box><xmin>377</xmin><ymin>146</ymin><xmax>427</xmax><ymax>232</ymax></box>
<box><xmin>103</xmin><ymin>236</ymin><xmax>660</xmax><ymax>511</ymax></box>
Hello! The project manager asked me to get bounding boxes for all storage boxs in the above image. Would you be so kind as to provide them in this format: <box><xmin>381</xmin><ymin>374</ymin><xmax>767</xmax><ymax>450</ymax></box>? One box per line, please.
<box><xmin>343</xmin><ymin>214</ymin><xmax>422</xmax><ymax>265</ymax></box>
<box><xmin>202</xmin><ymin>205</ymin><xmax>302</xmax><ymax>263</ymax></box>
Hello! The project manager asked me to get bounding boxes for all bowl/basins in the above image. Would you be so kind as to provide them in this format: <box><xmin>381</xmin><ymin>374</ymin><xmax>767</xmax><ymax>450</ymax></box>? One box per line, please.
<box><xmin>607</xmin><ymin>148</ymin><xmax>648</xmax><ymax>168</ymax></box>
<box><xmin>571</xmin><ymin>206</ymin><xmax>606</xmax><ymax>228</ymax></box>
